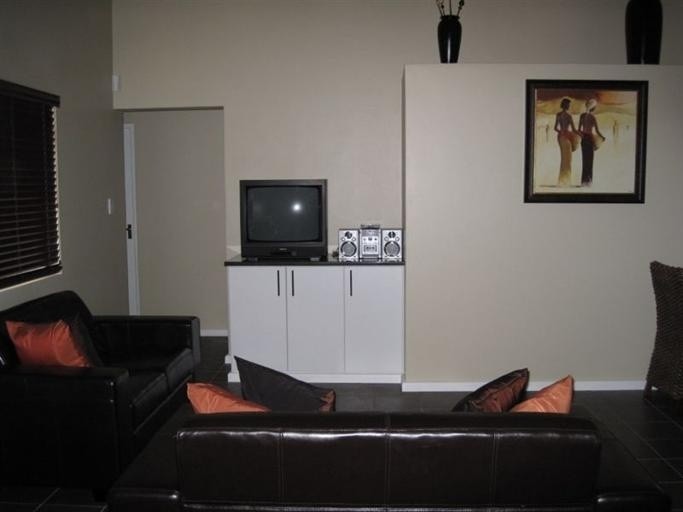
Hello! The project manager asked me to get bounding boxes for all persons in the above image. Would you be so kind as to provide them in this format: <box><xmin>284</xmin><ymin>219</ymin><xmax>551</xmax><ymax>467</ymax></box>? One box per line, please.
<box><xmin>577</xmin><ymin>97</ymin><xmax>607</xmax><ymax>184</ymax></box>
<box><xmin>554</xmin><ymin>97</ymin><xmax>578</xmax><ymax>184</ymax></box>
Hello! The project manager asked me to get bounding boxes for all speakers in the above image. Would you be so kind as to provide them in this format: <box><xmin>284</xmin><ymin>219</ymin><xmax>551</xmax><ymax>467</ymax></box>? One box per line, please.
<box><xmin>381</xmin><ymin>229</ymin><xmax>402</xmax><ymax>261</ymax></box>
<box><xmin>338</xmin><ymin>228</ymin><xmax>359</xmax><ymax>262</ymax></box>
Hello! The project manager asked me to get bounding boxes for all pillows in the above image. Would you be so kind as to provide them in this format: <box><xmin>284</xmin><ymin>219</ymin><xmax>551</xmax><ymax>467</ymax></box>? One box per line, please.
<box><xmin>449</xmin><ymin>368</ymin><xmax>529</xmax><ymax>413</ymax></box>
<box><xmin>61</xmin><ymin>310</ymin><xmax>103</xmax><ymax>368</ymax></box>
<box><xmin>510</xmin><ymin>374</ymin><xmax>574</xmax><ymax>415</ymax></box>
<box><xmin>233</xmin><ymin>355</ymin><xmax>337</xmax><ymax>414</ymax></box>
<box><xmin>186</xmin><ymin>381</ymin><xmax>272</xmax><ymax>413</ymax></box>
<box><xmin>6</xmin><ymin>319</ymin><xmax>92</xmax><ymax>369</ymax></box>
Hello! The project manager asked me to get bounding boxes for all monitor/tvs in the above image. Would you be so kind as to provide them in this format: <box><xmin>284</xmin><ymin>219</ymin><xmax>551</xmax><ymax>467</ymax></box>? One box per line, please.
<box><xmin>240</xmin><ymin>180</ymin><xmax>328</xmax><ymax>257</ymax></box>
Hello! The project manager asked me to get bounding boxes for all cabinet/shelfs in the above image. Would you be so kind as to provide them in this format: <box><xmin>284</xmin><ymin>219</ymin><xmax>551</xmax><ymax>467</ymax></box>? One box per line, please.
<box><xmin>227</xmin><ymin>265</ymin><xmax>345</xmax><ymax>375</ymax></box>
<box><xmin>344</xmin><ymin>265</ymin><xmax>404</xmax><ymax>376</ymax></box>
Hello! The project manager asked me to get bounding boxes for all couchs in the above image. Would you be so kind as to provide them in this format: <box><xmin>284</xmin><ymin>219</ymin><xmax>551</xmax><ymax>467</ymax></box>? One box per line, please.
<box><xmin>105</xmin><ymin>400</ymin><xmax>662</xmax><ymax>512</ymax></box>
<box><xmin>1</xmin><ymin>289</ymin><xmax>201</xmax><ymax>480</ymax></box>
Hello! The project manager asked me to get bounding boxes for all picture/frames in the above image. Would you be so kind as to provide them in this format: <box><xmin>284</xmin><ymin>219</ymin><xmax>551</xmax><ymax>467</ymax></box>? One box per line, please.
<box><xmin>523</xmin><ymin>80</ymin><xmax>647</xmax><ymax>204</ymax></box>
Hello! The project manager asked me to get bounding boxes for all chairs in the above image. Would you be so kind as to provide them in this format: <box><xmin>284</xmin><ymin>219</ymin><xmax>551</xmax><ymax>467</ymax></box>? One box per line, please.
<box><xmin>642</xmin><ymin>262</ymin><xmax>682</xmax><ymax>408</ymax></box>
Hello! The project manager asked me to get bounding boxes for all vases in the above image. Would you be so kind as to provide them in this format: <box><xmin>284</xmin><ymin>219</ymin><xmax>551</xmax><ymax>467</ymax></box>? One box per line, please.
<box><xmin>437</xmin><ymin>15</ymin><xmax>462</xmax><ymax>64</ymax></box>
<box><xmin>624</xmin><ymin>1</ymin><xmax>663</xmax><ymax>65</ymax></box>
